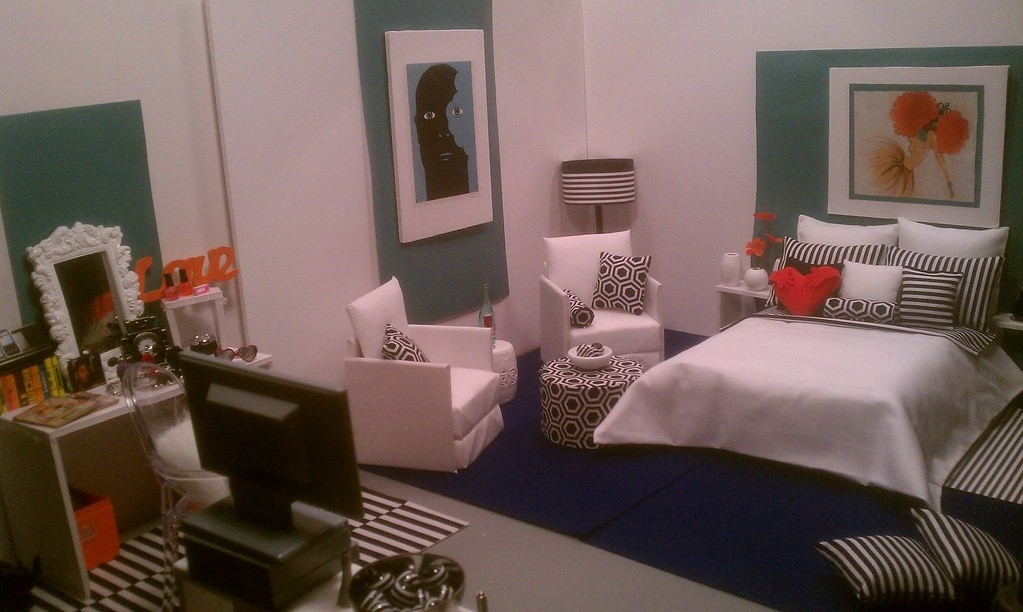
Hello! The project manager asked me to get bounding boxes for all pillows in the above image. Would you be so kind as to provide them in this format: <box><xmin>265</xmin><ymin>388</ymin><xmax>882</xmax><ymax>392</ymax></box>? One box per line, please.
<box><xmin>816</xmin><ymin>506</ymin><xmax>1023</xmax><ymax>602</ymax></box>
<box><xmin>564</xmin><ymin>251</ymin><xmax>652</xmax><ymax>327</ymax></box>
<box><xmin>766</xmin><ymin>236</ymin><xmax>1005</xmax><ymax>332</ymax></box>
<box><xmin>384</xmin><ymin>324</ymin><xmax>431</xmax><ymax>363</ymax></box>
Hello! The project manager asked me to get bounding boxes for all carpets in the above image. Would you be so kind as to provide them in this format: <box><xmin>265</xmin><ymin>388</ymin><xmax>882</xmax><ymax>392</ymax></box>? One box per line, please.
<box><xmin>944</xmin><ymin>406</ymin><xmax>1023</xmax><ymax>507</ymax></box>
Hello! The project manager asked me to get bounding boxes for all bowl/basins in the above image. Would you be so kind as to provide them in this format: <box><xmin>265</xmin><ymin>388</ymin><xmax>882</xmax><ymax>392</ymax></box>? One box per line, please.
<box><xmin>567</xmin><ymin>346</ymin><xmax>612</xmax><ymax>369</ymax></box>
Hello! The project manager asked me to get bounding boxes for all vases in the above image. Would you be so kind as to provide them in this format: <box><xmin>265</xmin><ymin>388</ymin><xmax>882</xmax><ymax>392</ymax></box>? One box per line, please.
<box><xmin>720</xmin><ymin>253</ymin><xmax>770</xmax><ymax>292</ymax></box>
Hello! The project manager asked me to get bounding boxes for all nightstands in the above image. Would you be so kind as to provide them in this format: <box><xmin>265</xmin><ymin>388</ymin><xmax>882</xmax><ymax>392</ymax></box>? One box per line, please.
<box><xmin>714</xmin><ymin>280</ymin><xmax>773</xmax><ymax>334</ymax></box>
<box><xmin>992</xmin><ymin>313</ymin><xmax>1023</xmax><ymax>344</ymax></box>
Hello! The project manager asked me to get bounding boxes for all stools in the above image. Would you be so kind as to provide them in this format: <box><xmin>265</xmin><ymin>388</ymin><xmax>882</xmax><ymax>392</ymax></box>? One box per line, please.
<box><xmin>492</xmin><ymin>340</ymin><xmax>643</xmax><ymax>450</ymax></box>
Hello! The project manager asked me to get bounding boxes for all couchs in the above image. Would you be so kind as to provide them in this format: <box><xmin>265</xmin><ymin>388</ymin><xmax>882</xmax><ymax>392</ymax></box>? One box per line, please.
<box><xmin>539</xmin><ymin>230</ymin><xmax>664</xmax><ymax>372</ymax></box>
<box><xmin>343</xmin><ymin>275</ymin><xmax>503</xmax><ymax>473</ymax></box>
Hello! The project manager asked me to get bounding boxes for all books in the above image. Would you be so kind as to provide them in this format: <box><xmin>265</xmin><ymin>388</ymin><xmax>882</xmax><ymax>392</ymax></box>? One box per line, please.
<box><xmin>12</xmin><ymin>396</ymin><xmax>97</xmax><ymax>429</ymax></box>
<box><xmin>0</xmin><ymin>355</ymin><xmax>66</xmax><ymax>413</ymax></box>
<box><xmin>68</xmin><ymin>389</ymin><xmax>120</xmax><ymax>410</ymax></box>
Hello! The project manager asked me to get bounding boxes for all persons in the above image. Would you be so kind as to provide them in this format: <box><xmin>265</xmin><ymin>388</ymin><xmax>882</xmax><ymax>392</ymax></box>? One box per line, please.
<box><xmin>73</xmin><ymin>359</ymin><xmax>93</xmax><ymax>390</ymax></box>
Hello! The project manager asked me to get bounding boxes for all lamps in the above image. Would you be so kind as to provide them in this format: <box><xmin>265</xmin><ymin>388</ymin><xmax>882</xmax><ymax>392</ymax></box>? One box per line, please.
<box><xmin>562</xmin><ymin>158</ymin><xmax>636</xmax><ymax>234</ymax></box>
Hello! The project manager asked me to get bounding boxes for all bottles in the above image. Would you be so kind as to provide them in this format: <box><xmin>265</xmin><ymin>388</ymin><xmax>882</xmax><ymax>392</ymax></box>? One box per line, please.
<box><xmin>178</xmin><ymin>268</ymin><xmax>193</xmax><ymax>296</ymax></box>
<box><xmin>478</xmin><ymin>282</ymin><xmax>496</xmax><ymax>345</ymax></box>
<box><xmin>163</xmin><ymin>273</ymin><xmax>179</xmax><ymax>300</ymax></box>
<box><xmin>720</xmin><ymin>252</ymin><xmax>741</xmax><ymax>287</ymax></box>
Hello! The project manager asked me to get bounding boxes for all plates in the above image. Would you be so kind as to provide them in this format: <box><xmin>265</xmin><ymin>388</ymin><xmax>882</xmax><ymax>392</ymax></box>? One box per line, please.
<box><xmin>348</xmin><ymin>553</ymin><xmax>466</xmax><ymax>611</ymax></box>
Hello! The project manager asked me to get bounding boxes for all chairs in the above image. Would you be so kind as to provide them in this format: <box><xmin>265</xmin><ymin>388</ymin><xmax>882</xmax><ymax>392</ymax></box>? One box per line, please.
<box><xmin>123</xmin><ymin>362</ymin><xmax>236</xmax><ymax>612</ymax></box>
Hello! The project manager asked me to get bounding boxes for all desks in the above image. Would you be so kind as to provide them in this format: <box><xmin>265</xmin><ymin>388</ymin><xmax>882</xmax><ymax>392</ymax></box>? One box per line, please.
<box><xmin>0</xmin><ymin>348</ymin><xmax>273</xmax><ymax>599</ymax></box>
<box><xmin>172</xmin><ymin>472</ymin><xmax>776</xmax><ymax>612</ymax></box>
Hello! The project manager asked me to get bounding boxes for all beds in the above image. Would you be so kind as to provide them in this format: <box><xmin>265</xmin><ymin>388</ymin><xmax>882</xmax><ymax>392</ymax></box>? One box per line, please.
<box><xmin>594</xmin><ymin>215</ymin><xmax>1023</xmax><ymax>514</ymax></box>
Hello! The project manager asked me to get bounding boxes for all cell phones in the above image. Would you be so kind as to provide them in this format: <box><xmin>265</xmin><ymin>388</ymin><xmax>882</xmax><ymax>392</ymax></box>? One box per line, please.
<box><xmin>0</xmin><ymin>329</ymin><xmax>22</xmax><ymax>358</ymax></box>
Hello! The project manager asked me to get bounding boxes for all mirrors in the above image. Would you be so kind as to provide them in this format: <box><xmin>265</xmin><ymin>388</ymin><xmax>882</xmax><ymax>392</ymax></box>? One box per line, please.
<box><xmin>27</xmin><ymin>221</ymin><xmax>144</xmax><ymax>392</ymax></box>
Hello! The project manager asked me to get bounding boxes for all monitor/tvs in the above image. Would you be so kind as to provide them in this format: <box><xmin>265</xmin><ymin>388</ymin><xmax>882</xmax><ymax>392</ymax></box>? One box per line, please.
<box><xmin>181</xmin><ymin>349</ymin><xmax>364</xmax><ymax>565</ymax></box>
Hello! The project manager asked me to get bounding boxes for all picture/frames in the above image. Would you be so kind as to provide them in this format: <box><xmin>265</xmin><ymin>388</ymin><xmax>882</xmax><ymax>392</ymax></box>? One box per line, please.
<box><xmin>828</xmin><ymin>65</ymin><xmax>1007</xmax><ymax>227</ymax></box>
<box><xmin>384</xmin><ymin>29</ymin><xmax>493</xmax><ymax>244</ymax></box>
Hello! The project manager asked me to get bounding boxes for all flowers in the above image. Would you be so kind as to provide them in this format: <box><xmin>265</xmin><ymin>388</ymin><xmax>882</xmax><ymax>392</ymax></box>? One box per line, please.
<box><xmin>745</xmin><ymin>212</ymin><xmax>783</xmax><ymax>270</ymax></box>
